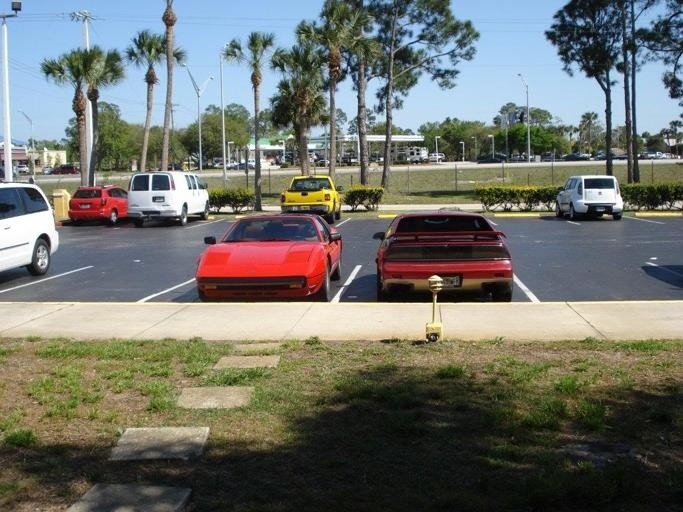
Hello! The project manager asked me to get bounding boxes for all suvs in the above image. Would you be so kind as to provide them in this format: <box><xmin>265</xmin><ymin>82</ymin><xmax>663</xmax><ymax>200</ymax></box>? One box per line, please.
<box><xmin>554</xmin><ymin>175</ymin><xmax>625</xmax><ymax>220</ymax></box>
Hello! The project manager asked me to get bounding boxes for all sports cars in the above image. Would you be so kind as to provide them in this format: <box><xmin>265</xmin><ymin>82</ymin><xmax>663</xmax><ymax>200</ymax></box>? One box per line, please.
<box><xmin>196</xmin><ymin>212</ymin><xmax>344</xmax><ymax>303</ymax></box>
<box><xmin>371</xmin><ymin>210</ymin><xmax>515</xmax><ymax>300</ymax></box>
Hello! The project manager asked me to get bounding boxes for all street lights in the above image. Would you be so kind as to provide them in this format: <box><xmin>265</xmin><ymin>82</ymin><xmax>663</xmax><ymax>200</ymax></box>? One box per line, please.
<box><xmin>434</xmin><ymin>136</ymin><xmax>441</xmax><ymax>162</ymax></box>
<box><xmin>486</xmin><ymin>135</ymin><xmax>495</xmax><ymax>160</ymax></box>
<box><xmin>227</xmin><ymin>141</ymin><xmax>234</xmax><ymax>169</ymax></box>
<box><xmin>277</xmin><ymin>140</ymin><xmax>285</xmax><ymax>163</ymax></box>
<box><xmin>518</xmin><ymin>73</ymin><xmax>530</xmax><ymax>162</ymax></box>
<box><xmin>181</xmin><ymin>62</ymin><xmax>215</xmax><ymax>171</ymax></box>
<box><xmin>458</xmin><ymin>141</ymin><xmax>464</xmax><ymax>162</ymax></box>
<box><xmin>471</xmin><ymin>137</ymin><xmax>477</xmax><ymax>162</ymax></box>
<box><xmin>16</xmin><ymin>108</ymin><xmax>35</xmax><ymax>175</ymax></box>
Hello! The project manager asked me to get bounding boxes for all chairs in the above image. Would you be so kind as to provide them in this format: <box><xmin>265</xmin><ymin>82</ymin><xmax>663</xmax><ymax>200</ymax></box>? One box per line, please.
<box><xmin>242</xmin><ymin>226</ymin><xmax>265</xmax><ymax>240</ymax></box>
<box><xmin>297</xmin><ymin>225</ymin><xmax>316</xmax><ymax>238</ymax></box>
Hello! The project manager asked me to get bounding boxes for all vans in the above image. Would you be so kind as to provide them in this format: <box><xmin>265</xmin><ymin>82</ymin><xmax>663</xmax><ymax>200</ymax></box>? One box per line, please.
<box><xmin>126</xmin><ymin>169</ymin><xmax>212</xmax><ymax>227</ymax></box>
<box><xmin>0</xmin><ymin>178</ymin><xmax>59</xmax><ymax>278</ymax></box>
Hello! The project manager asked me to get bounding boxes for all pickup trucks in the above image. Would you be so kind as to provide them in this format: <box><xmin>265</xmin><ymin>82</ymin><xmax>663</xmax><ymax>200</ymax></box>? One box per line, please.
<box><xmin>280</xmin><ymin>174</ymin><xmax>344</xmax><ymax>223</ymax></box>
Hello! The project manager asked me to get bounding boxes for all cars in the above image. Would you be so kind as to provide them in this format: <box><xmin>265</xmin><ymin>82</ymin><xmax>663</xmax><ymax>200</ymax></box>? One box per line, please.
<box><xmin>157</xmin><ymin>157</ymin><xmax>256</xmax><ymax>173</ymax></box>
<box><xmin>67</xmin><ymin>184</ymin><xmax>129</xmax><ymax>226</ymax></box>
<box><xmin>272</xmin><ymin>146</ymin><xmax>459</xmax><ymax>166</ymax></box>
<box><xmin>475</xmin><ymin>148</ymin><xmax>683</xmax><ymax>163</ymax></box>
<box><xmin>0</xmin><ymin>164</ymin><xmax>81</xmax><ymax>178</ymax></box>
